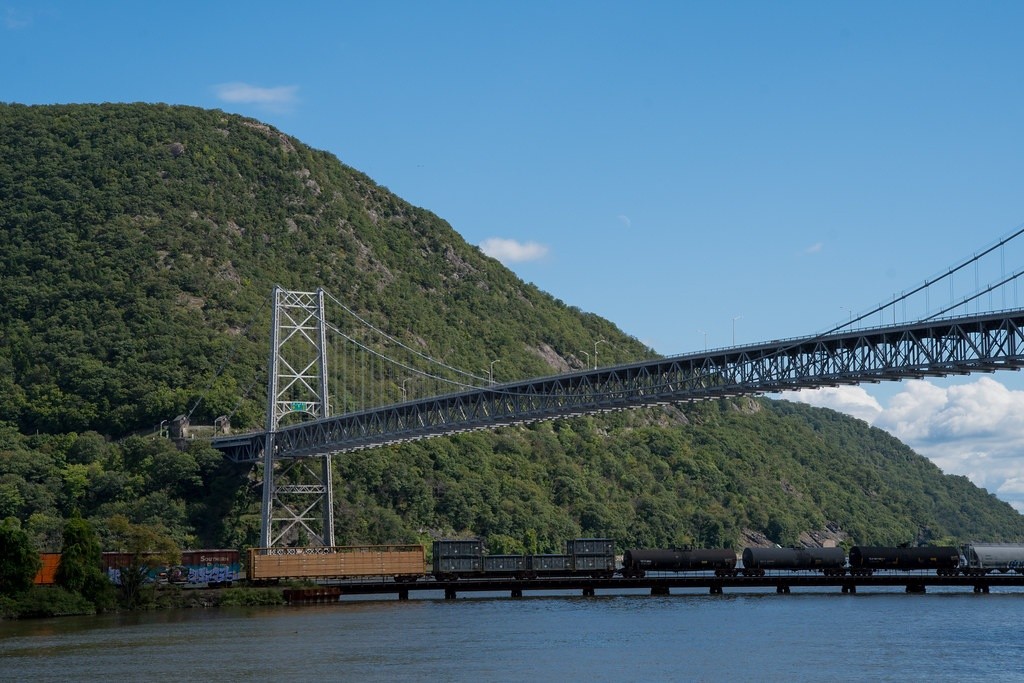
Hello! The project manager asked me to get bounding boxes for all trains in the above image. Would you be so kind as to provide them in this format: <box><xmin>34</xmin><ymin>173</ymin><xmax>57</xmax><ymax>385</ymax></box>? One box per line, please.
<box><xmin>29</xmin><ymin>539</ymin><xmax>1023</xmax><ymax>584</ymax></box>
